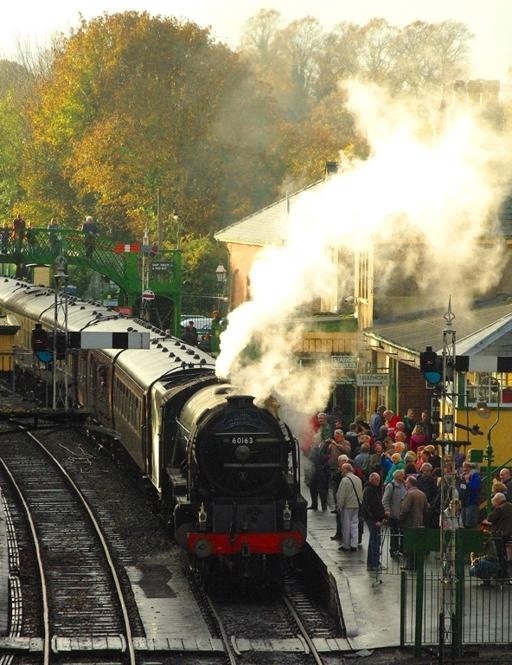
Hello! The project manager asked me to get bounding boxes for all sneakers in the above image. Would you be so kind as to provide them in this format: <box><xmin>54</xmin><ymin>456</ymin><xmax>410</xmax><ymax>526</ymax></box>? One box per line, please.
<box><xmin>350</xmin><ymin>547</ymin><xmax>358</xmax><ymax>552</ymax></box>
<box><xmin>338</xmin><ymin>546</ymin><xmax>349</xmax><ymax>552</ymax></box>
<box><xmin>368</xmin><ymin>564</ymin><xmax>387</xmax><ymax>571</ymax></box>
<box><xmin>441</xmin><ymin>575</ymin><xmax>453</xmax><ymax>585</ymax></box>
<box><xmin>400</xmin><ymin>565</ymin><xmax>415</xmax><ymax>570</ymax></box>
<box><xmin>331</xmin><ymin>511</ymin><xmax>337</xmax><ymax>514</ymax></box>
<box><xmin>307</xmin><ymin>506</ymin><xmax>318</xmax><ymax>510</ymax></box>
<box><xmin>330</xmin><ymin>534</ymin><xmax>343</xmax><ymax>540</ymax></box>
<box><xmin>322</xmin><ymin>504</ymin><xmax>327</xmax><ymax>510</ymax></box>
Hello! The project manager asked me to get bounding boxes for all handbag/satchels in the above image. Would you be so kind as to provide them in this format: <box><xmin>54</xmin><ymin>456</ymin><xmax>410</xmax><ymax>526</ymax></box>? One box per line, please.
<box><xmin>422</xmin><ymin>511</ymin><xmax>436</xmax><ymax>528</ymax></box>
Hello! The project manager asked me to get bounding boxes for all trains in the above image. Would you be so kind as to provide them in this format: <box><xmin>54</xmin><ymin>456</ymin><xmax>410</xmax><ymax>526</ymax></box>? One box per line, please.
<box><xmin>0</xmin><ymin>275</ymin><xmax>308</xmax><ymax>577</ymax></box>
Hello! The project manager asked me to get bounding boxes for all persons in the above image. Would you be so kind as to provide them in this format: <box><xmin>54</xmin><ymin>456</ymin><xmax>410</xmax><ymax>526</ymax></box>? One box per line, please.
<box><xmin>9</xmin><ymin>212</ymin><xmax>27</xmax><ymax>256</ymax></box>
<box><xmin>79</xmin><ymin>215</ymin><xmax>100</xmax><ymax>259</ymax></box>
<box><xmin>298</xmin><ymin>404</ymin><xmax>510</xmax><ymax>591</ymax></box>
<box><xmin>24</xmin><ymin>220</ymin><xmax>40</xmax><ymax>254</ymax></box>
<box><xmin>0</xmin><ymin>220</ymin><xmax>10</xmax><ymax>256</ymax></box>
<box><xmin>179</xmin><ymin>309</ymin><xmax>222</xmax><ymax>353</ymax></box>
<box><xmin>45</xmin><ymin>215</ymin><xmax>63</xmax><ymax>256</ymax></box>
